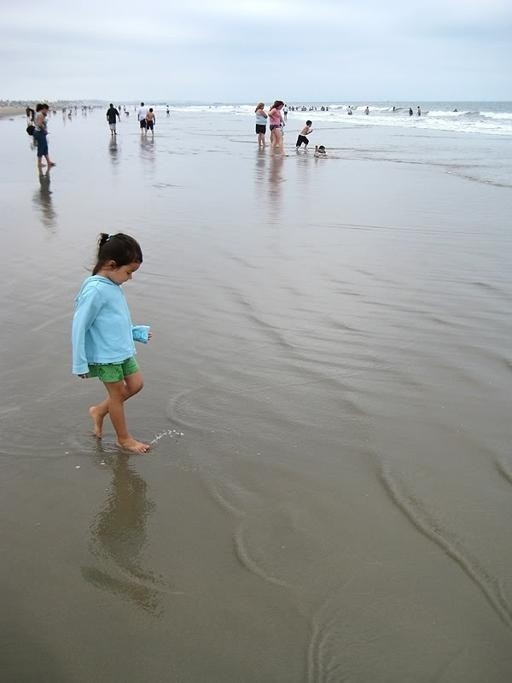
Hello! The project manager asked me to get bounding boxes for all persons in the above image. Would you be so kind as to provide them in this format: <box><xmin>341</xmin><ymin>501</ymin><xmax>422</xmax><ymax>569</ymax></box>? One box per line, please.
<box><xmin>270</xmin><ymin>103</ymin><xmax>284</xmax><ymax>136</ymax></box>
<box><xmin>347</xmin><ymin>105</ymin><xmax>352</xmax><ymax>114</ymax></box>
<box><xmin>72</xmin><ymin>231</ymin><xmax>153</xmax><ymax>454</ymax></box>
<box><xmin>409</xmin><ymin>107</ymin><xmax>413</xmax><ymax>115</ymax></box>
<box><xmin>288</xmin><ymin>105</ymin><xmax>329</xmax><ymax>111</ymax></box>
<box><xmin>34</xmin><ymin>103</ymin><xmax>57</xmax><ymax>166</ymax></box>
<box><xmin>106</xmin><ymin>102</ymin><xmax>121</xmax><ymax>134</ymax></box>
<box><xmin>30</xmin><ymin>107</ymin><xmax>35</xmax><ymax>125</ymax></box>
<box><xmin>145</xmin><ymin>106</ymin><xmax>156</xmax><ymax>133</ymax></box>
<box><xmin>138</xmin><ymin>101</ymin><xmax>148</xmax><ymax>134</ymax></box>
<box><xmin>417</xmin><ymin>105</ymin><xmax>421</xmax><ymax>115</ymax></box>
<box><xmin>27</xmin><ymin>105</ymin><xmax>30</xmax><ymax>119</ymax></box>
<box><xmin>269</xmin><ymin>100</ymin><xmax>285</xmax><ymax>148</ymax></box>
<box><xmin>295</xmin><ymin>119</ymin><xmax>314</xmax><ymax>150</ymax></box>
<box><xmin>31</xmin><ymin>103</ymin><xmax>43</xmax><ymax>148</ymax></box>
<box><xmin>314</xmin><ymin>144</ymin><xmax>326</xmax><ymax>153</ymax></box>
<box><xmin>283</xmin><ymin>104</ymin><xmax>288</xmax><ymax>119</ymax></box>
<box><xmin>365</xmin><ymin>106</ymin><xmax>369</xmax><ymax>114</ymax></box>
<box><xmin>254</xmin><ymin>102</ymin><xmax>268</xmax><ymax>147</ymax></box>
<box><xmin>50</xmin><ymin>103</ymin><xmax>137</xmax><ymax>116</ymax></box>
<box><xmin>166</xmin><ymin>104</ymin><xmax>170</xmax><ymax>115</ymax></box>
